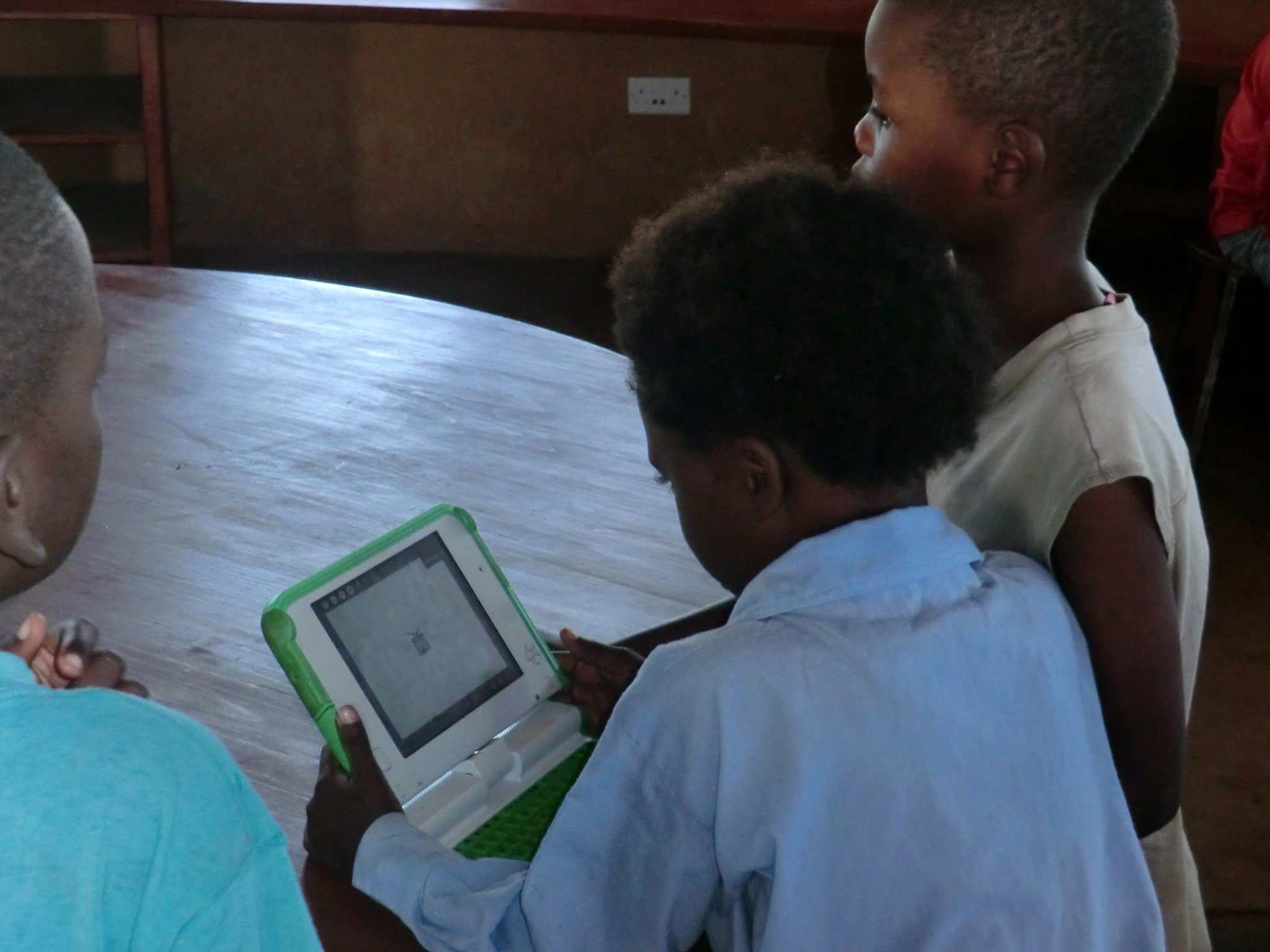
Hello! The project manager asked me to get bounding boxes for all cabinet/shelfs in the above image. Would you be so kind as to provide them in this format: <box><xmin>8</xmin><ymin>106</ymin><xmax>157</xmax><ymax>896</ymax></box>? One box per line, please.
<box><xmin>0</xmin><ymin>0</ymin><xmax>176</xmax><ymax>270</ymax></box>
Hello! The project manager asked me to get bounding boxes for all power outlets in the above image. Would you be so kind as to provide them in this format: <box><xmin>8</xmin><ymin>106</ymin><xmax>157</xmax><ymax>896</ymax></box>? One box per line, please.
<box><xmin>627</xmin><ymin>76</ymin><xmax>692</xmax><ymax>116</ymax></box>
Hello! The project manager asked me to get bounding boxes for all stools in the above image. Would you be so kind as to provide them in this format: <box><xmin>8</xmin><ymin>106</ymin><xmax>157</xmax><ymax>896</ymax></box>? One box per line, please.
<box><xmin>1181</xmin><ymin>220</ymin><xmax>1256</xmax><ymax>470</ymax></box>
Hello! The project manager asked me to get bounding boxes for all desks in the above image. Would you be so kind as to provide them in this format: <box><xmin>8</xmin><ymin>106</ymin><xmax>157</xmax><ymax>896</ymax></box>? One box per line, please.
<box><xmin>1</xmin><ymin>261</ymin><xmax>734</xmax><ymax>952</ymax></box>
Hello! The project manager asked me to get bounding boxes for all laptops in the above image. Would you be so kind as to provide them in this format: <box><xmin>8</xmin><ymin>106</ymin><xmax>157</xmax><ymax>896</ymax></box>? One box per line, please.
<box><xmin>260</xmin><ymin>501</ymin><xmax>602</xmax><ymax>863</ymax></box>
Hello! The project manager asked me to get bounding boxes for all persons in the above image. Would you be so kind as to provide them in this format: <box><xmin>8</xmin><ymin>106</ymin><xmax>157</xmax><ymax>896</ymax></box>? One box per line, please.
<box><xmin>303</xmin><ymin>158</ymin><xmax>1176</xmax><ymax>952</ymax></box>
<box><xmin>853</xmin><ymin>0</ymin><xmax>1270</xmax><ymax>952</ymax></box>
<box><xmin>0</xmin><ymin>131</ymin><xmax>330</xmax><ymax>951</ymax></box>
